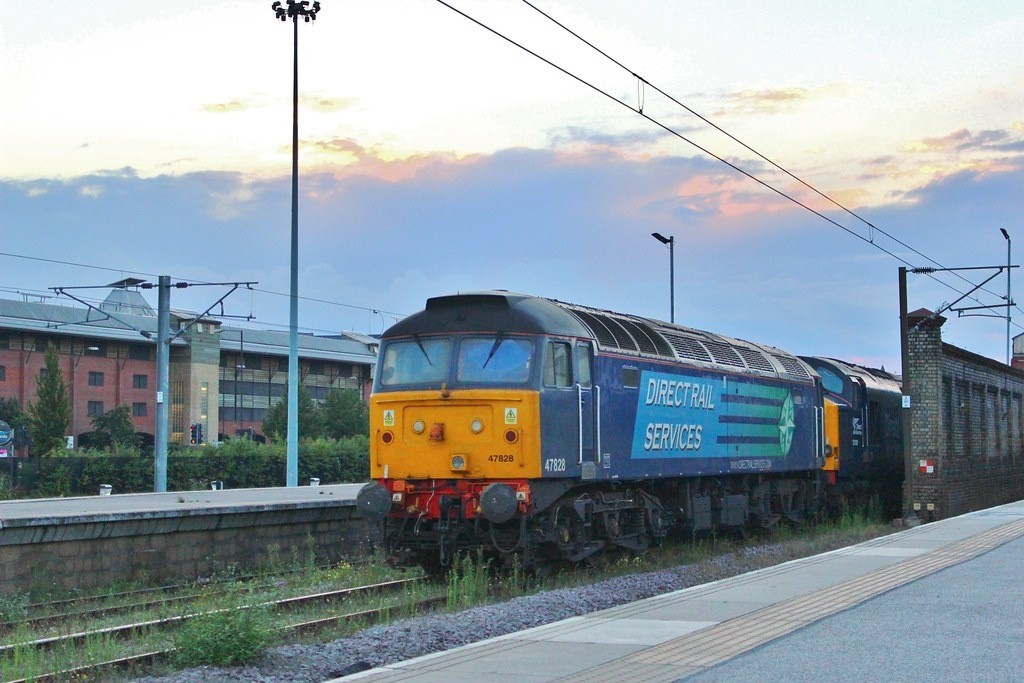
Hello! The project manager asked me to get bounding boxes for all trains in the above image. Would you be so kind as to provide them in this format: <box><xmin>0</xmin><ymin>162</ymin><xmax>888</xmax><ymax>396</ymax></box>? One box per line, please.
<box><xmin>357</xmin><ymin>289</ymin><xmax>905</xmax><ymax>579</ymax></box>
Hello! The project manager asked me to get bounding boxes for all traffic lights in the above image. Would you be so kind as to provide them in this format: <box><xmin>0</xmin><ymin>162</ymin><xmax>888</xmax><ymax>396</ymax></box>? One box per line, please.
<box><xmin>189</xmin><ymin>425</ymin><xmax>197</xmax><ymax>445</ymax></box>
<box><xmin>196</xmin><ymin>423</ymin><xmax>204</xmax><ymax>444</ymax></box>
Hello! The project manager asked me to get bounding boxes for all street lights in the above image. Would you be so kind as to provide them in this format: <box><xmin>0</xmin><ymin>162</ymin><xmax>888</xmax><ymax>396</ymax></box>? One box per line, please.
<box><xmin>213</xmin><ymin>328</ymin><xmax>244</xmax><ymax>439</ymax></box>
<box><xmin>651</xmin><ymin>231</ymin><xmax>675</xmax><ymax>324</ymax></box>
<box><xmin>1000</xmin><ymin>227</ymin><xmax>1012</xmax><ymax>366</ymax></box>
<box><xmin>271</xmin><ymin>0</ymin><xmax>323</xmax><ymax>488</ymax></box>
